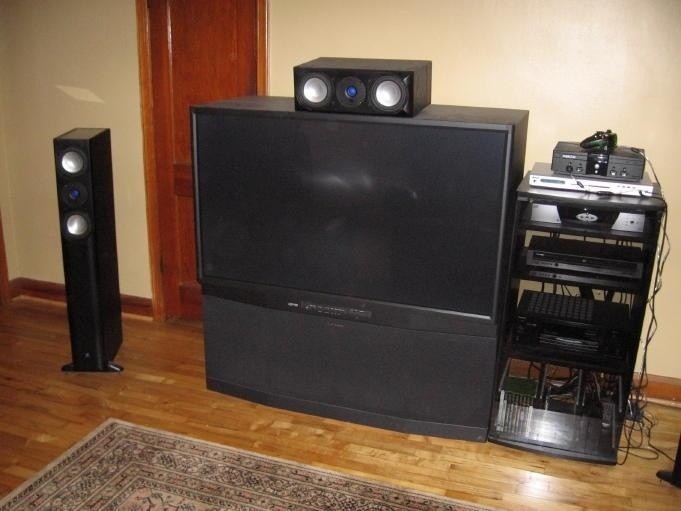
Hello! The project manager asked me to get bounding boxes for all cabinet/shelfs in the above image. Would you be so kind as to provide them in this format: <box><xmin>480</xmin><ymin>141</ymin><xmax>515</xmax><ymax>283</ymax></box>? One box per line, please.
<box><xmin>499</xmin><ymin>166</ymin><xmax>665</xmax><ymax>466</ymax></box>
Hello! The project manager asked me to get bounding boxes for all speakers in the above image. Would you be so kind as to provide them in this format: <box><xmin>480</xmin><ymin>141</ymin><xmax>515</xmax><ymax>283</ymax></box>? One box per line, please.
<box><xmin>293</xmin><ymin>57</ymin><xmax>432</xmax><ymax>118</ymax></box>
<box><xmin>53</xmin><ymin>129</ymin><xmax>124</xmax><ymax>373</ymax></box>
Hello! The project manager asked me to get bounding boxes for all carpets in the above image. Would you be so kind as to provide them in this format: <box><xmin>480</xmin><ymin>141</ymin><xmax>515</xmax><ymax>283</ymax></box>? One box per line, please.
<box><xmin>1</xmin><ymin>418</ymin><xmax>493</xmax><ymax>511</ymax></box>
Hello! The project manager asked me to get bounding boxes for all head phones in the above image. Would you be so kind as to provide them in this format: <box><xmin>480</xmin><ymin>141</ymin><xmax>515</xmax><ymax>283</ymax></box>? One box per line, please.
<box><xmin>580</xmin><ymin>128</ymin><xmax>617</xmax><ymax>151</ymax></box>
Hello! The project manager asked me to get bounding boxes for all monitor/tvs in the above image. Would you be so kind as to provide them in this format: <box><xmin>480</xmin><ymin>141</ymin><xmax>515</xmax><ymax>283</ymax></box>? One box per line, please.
<box><xmin>190</xmin><ymin>96</ymin><xmax>528</xmax><ymax>338</ymax></box>
